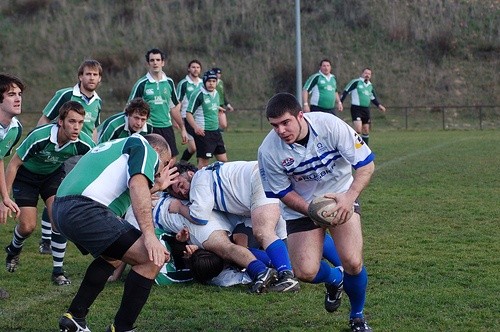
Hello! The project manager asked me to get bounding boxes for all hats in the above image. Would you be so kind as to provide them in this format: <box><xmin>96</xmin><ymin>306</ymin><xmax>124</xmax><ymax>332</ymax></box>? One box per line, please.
<box><xmin>203</xmin><ymin>70</ymin><xmax>217</xmax><ymax>86</ymax></box>
<box><xmin>212</xmin><ymin>68</ymin><xmax>222</xmax><ymax>74</ymax></box>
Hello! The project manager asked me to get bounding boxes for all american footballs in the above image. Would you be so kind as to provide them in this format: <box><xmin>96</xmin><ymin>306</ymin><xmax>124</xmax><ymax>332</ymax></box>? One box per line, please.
<box><xmin>307</xmin><ymin>193</ymin><xmax>355</xmax><ymax>228</ymax></box>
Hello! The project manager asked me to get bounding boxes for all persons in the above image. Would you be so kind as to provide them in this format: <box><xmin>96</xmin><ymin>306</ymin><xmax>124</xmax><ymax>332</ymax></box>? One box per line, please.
<box><xmin>1</xmin><ymin>101</ymin><xmax>98</xmax><ymax>286</ymax></box>
<box><xmin>0</xmin><ymin>72</ymin><xmax>23</xmax><ymax>220</ymax></box>
<box><xmin>96</xmin><ymin>98</ymin><xmax>150</xmax><ymax>145</ymax></box>
<box><xmin>173</xmin><ymin>60</ymin><xmax>204</xmax><ymax>162</ymax></box>
<box><xmin>302</xmin><ymin>59</ymin><xmax>343</xmax><ymax>116</ymax></box>
<box><xmin>107</xmin><ymin>162</ymin><xmax>343</xmax><ymax>293</ymax></box>
<box><xmin>340</xmin><ymin>68</ymin><xmax>386</xmax><ymax>145</ymax></box>
<box><xmin>123</xmin><ymin>49</ymin><xmax>188</xmax><ymax>162</ymax></box>
<box><xmin>52</xmin><ymin>133</ymin><xmax>180</xmax><ymax>332</ymax></box>
<box><xmin>257</xmin><ymin>93</ymin><xmax>376</xmax><ymax>332</ymax></box>
<box><xmin>211</xmin><ymin>67</ymin><xmax>233</xmax><ymax>136</ymax></box>
<box><xmin>36</xmin><ymin>60</ymin><xmax>103</xmax><ymax>255</ymax></box>
<box><xmin>186</xmin><ymin>71</ymin><xmax>228</xmax><ymax>170</ymax></box>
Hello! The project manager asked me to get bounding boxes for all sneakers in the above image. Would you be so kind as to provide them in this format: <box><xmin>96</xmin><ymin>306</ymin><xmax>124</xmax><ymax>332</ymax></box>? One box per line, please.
<box><xmin>106</xmin><ymin>324</ymin><xmax>137</xmax><ymax>332</ymax></box>
<box><xmin>349</xmin><ymin>316</ymin><xmax>371</xmax><ymax>332</ymax></box>
<box><xmin>59</xmin><ymin>312</ymin><xmax>91</xmax><ymax>332</ymax></box>
<box><xmin>108</xmin><ymin>261</ymin><xmax>127</xmax><ymax>281</ymax></box>
<box><xmin>52</xmin><ymin>272</ymin><xmax>70</xmax><ymax>284</ymax></box>
<box><xmin>269</xmin><ymin>271</ymin><xmax>300</xmax><ymax>291</ymax></box>
<box><xmin>5</xmin><ymin>242</ymin><xmax>20</xmax><ymax>273</ymax></box>
<box><xmin>252</xmin><ymin>267</ymin><xmax>277</xmax><ymax>293</ymax></box>
<box><xmin>39</xmin><ymin>241</ymin><xmax>52</xmax><ymax>254</ymax></box>
<box><xmin>325</xmin><ymin>266</ymin><xmax>344</xmax><ymax>313</ymax></box>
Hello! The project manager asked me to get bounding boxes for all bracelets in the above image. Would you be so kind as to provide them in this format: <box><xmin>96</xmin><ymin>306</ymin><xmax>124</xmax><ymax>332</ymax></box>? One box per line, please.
<box><xmin>338</xmin><ymin>101</ymin><xmax>342</xmax><ymax>105</ymax></box>
<box><xmin>304</xmin><ymin>102</ymin><xmax>309</xmax><ymax>105</ymax></box>
